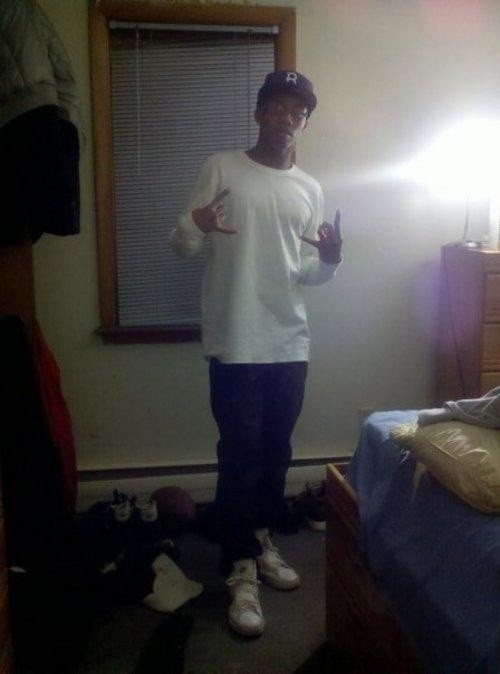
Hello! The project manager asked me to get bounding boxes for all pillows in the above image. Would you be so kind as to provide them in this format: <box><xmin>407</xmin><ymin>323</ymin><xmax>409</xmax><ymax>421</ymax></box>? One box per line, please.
<box><xmin>391</xmin><ymin>420</ymin><xmax>500</xmax><ymax>511</ymax></box>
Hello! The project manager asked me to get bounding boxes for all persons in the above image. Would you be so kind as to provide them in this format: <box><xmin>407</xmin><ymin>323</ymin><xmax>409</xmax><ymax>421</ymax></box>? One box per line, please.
<box><xmin>167</xmin><ymin>66</ymin><xmax>347</xmax><ymax>639</ymax></box>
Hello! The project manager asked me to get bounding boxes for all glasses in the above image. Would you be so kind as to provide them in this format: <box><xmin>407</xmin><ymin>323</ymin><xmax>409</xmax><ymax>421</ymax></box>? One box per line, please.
<box><xmin>262</xmin><ymin>102</ymin><xmax>314</xmax><ymax>121</ymax></box>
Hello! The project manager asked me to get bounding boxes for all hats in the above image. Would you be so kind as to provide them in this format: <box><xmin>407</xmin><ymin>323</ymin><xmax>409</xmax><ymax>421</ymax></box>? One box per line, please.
<box><xmin>257</xmin><ymin>71</ymin><xmax>317</xmax><ymax>105</ymax></box>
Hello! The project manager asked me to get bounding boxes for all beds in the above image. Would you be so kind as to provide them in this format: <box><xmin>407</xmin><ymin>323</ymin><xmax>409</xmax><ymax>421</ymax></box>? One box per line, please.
<box><xmin>323</xmin><ymin>407</ymin><xmax>500</xmax><ymax>671</ymax></box>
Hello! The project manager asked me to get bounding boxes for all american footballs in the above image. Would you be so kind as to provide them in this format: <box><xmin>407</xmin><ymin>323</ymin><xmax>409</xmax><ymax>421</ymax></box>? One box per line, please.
<box><xmin>152</xmin><ymin>486</ymin><xmax>196</xmax><ymax>524</ymax></box>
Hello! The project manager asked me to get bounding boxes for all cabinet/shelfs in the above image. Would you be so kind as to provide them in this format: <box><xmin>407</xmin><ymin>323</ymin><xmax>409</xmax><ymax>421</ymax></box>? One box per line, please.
<box><xmin>435</xmin><ymin>243</ymin><xmax>500</xmax><ymax>409</ymax></box>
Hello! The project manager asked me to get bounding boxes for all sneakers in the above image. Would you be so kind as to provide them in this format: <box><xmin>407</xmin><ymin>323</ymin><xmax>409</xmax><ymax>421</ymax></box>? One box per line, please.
<box><xmin>225</xmin><ymin>558</ymin><xmax>266</xmax><ymax>638</ymax></box>
<box><xmin>302</xmin><ymin>481</ymin><xmax>327</xmax><ymax>532</ymax></box>
<box><xmin>253</xmin><ymin>528</ymin><xmax>302</xmax><ymax>591</ymax></box>
